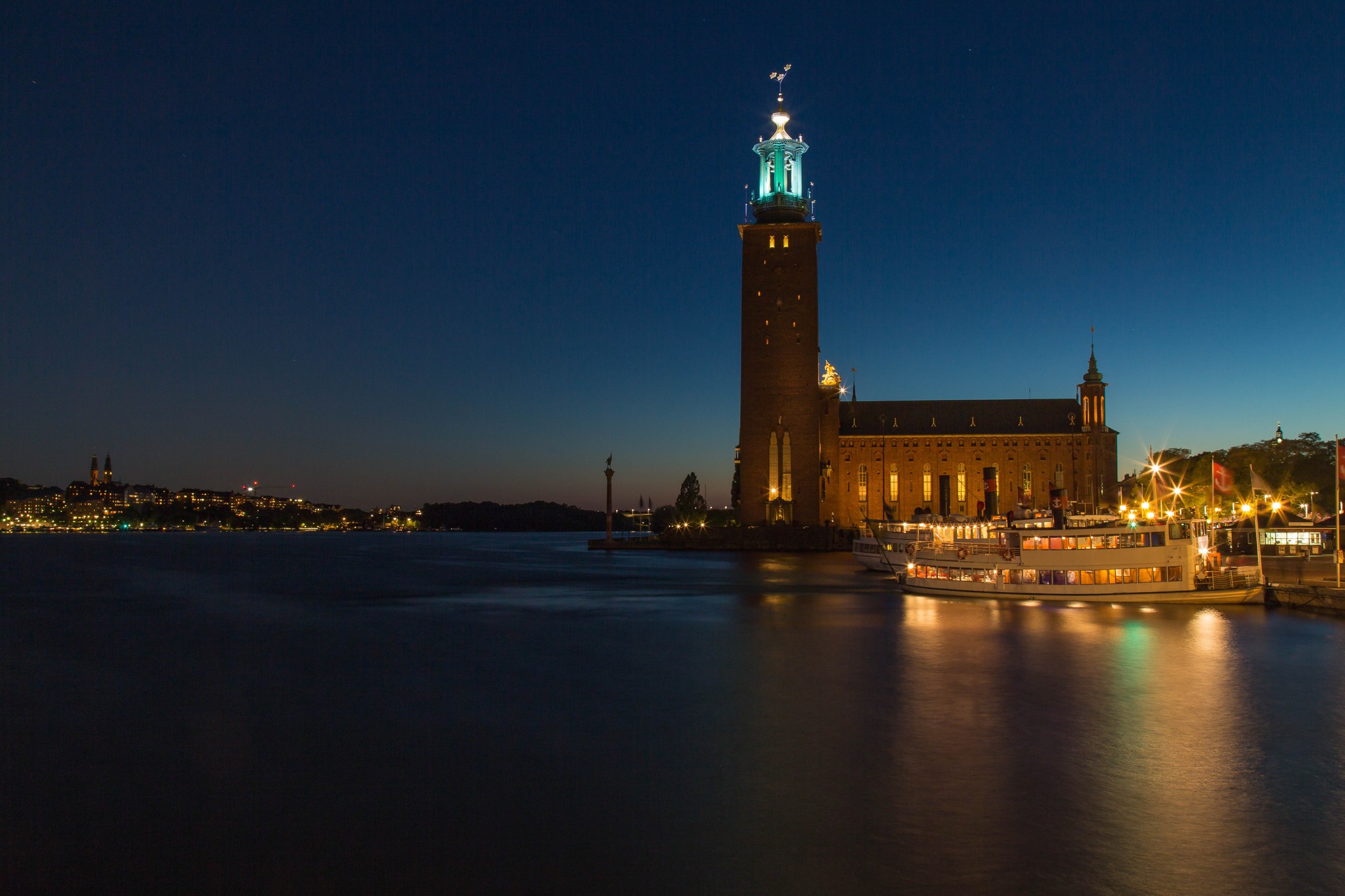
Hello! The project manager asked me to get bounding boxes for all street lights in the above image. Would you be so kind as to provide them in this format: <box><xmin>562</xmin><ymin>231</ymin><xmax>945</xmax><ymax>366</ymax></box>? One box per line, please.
<box><xmin>1310</xmin><ymin>492</ymin><xmax>1319</xmax><ymax>517</ymax></box>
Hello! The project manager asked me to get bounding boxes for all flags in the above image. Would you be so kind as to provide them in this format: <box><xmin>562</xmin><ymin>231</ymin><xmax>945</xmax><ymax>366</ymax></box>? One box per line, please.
<box><xmin>1337</xmin><ymin>440</ymin><xmax>1345</xmax><ymax>481</ymax></box>
<box><xmin>1213</xmin><ymin>461</ymin><xmax>1236</xmax><ymax>498</ymax></box>
<box><xmin>1251</xmin><ymin>468</ymin><xmax>1268</xmax><ymax>491</ymax></box>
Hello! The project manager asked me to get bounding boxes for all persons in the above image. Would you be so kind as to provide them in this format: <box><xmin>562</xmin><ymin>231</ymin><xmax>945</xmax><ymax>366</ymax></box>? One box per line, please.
<box><xmin>985</xmin><ymin>570</ymin><xmax>1076</xmax><ymax>585</ymax></box>
<box><xmin>1190</xmin><ymin>515</ymin><xmax>1202</xmax><ymax>538</ymax></box>
<box><xmin>928</xmin><ymin>567</ymin><xmax>978</xmax><ymax>581</ymax></box>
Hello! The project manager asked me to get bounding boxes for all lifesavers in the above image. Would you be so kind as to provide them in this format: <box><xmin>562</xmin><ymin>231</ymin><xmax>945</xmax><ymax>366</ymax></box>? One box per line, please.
<box><xmin>907</xmin><ymin>545</ymin><xmax>915</xmax><ymax>556</ymax></box>
<box><xmin>1003</xmin><ymin>549</ymin><xmax>1015</xmax><ymax>561</ymax></box>
<box><xmin>958</xmin><ymin>548</ymin><xmax>968</xmax><ymax>560</ymax></box>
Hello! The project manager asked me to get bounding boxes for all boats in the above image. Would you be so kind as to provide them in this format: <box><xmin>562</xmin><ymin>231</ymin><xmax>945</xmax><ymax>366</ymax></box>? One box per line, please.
<box><xmin>848</xmin><ymin>466</ymin><xmax>1270</xmax><ymax>604</ymax></box>
